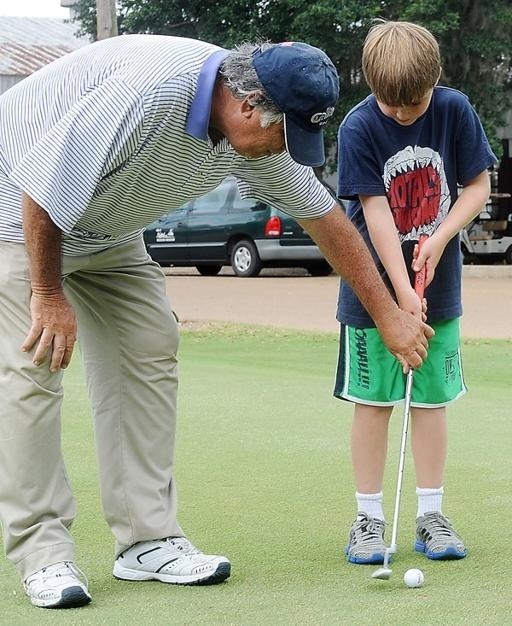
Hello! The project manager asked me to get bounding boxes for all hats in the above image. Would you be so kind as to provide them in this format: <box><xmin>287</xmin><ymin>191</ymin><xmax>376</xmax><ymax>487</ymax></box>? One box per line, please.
<box><xmin>252</xmin><ymin>42</ymin><xmax>341</xmax><ymax>167</ymax></box>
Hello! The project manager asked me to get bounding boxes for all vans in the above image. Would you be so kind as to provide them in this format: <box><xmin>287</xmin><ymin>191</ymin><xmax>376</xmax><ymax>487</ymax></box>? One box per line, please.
<box><xmin>144</xmin><ymin>175</ymin><xmax>348</xmax><ymax>276</ymax></box>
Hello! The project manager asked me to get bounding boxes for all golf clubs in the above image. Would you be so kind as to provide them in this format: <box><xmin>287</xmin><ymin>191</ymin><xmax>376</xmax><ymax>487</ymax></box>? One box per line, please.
<box><xmin>371</xmin><ymin>232</ymin><xmax>428</xmax><ymax>580</ymax></box>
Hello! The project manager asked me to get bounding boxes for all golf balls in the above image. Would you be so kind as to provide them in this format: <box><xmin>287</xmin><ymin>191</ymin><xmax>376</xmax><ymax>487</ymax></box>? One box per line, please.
<box><xmin>404</xmin><ymin>568</ymin><xmax>425</xmax><ymax>588</ymax></box>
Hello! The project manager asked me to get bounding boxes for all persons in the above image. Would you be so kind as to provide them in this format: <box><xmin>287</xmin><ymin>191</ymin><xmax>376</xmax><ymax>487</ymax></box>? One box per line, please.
<box><xmin>332</xmin><ymin>19</ymin><xmax>499</xmax><ymax>566</ymax></box>
<box><xmin>0</xmin><ymin>33</ymin><xmax>435</xmax><ymax>610</ymax></box>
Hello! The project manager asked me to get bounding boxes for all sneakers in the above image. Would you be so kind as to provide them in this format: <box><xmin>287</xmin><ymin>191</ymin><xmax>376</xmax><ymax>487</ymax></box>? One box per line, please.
<box><xmin>345</xmin><ymin>511</ymin><xmax>391</xmax><ymax>564</ymax></box>
<box><xmin>111</xmin><ymin>539</ymin><xmax>231</xmax><ymax>586</ymax></box>
<box><xmin>414</xmin><ymin>510</ymin><xmax>467</xmax><ymax>561</ymax></box>
<box><xmin>23</xmin><ymin>559</ymin><xmax>94</xmax><ymax>610</ymax></box>
